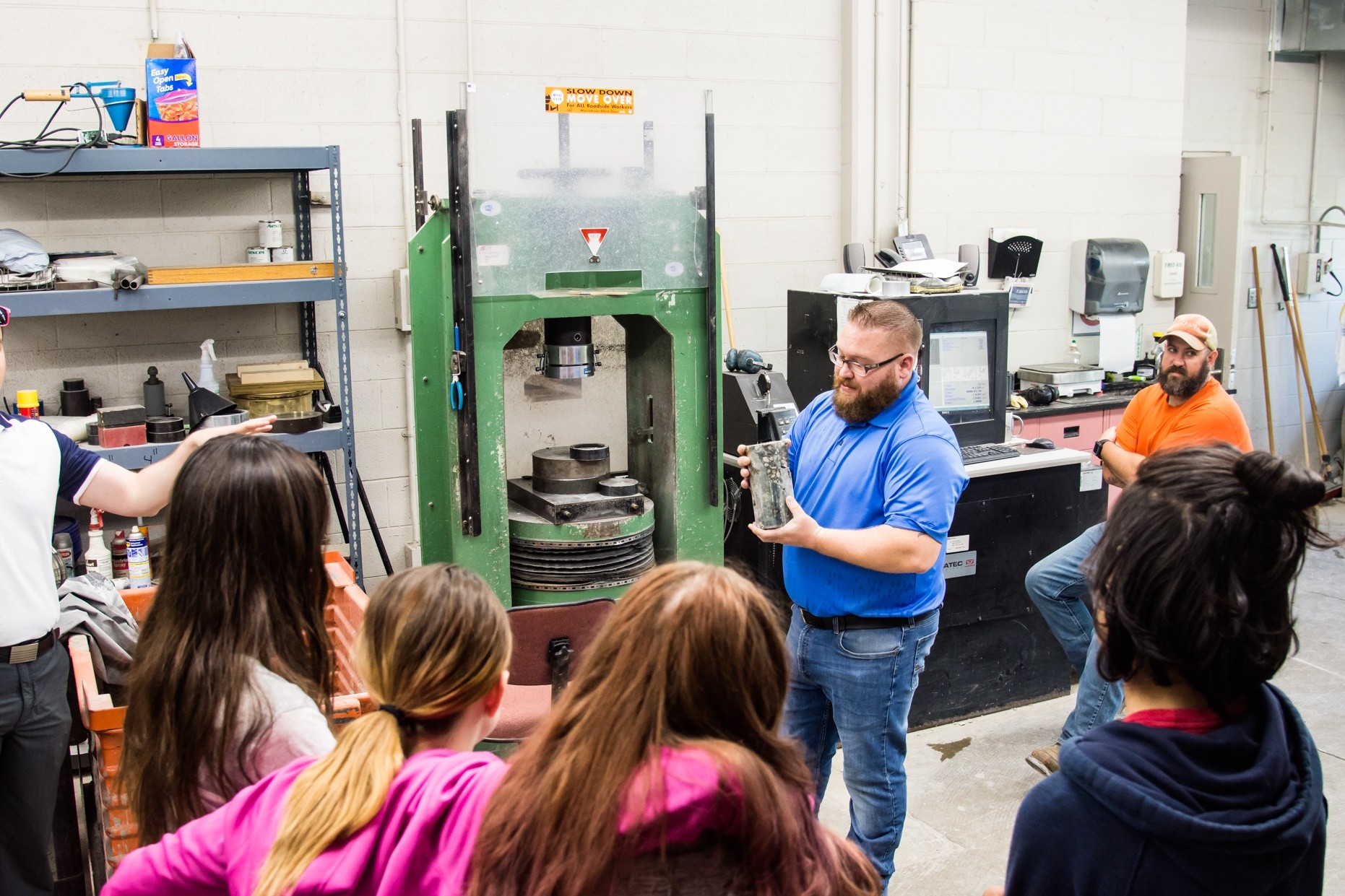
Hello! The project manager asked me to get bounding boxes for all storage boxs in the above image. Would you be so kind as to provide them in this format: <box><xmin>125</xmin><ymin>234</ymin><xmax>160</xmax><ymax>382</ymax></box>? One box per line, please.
<box><xmin>64</xmin><ymin>555</ymin><xmax>379</xmax><ymax>882</ymax></box>
<box><xmin>145</xmin><ymin>39</ymin><xmax>202</xmax><ymax>148</ymax></box>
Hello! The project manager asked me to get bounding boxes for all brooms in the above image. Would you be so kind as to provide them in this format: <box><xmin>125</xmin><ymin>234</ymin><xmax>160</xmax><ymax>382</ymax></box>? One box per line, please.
<box><xmin>1269</xmin><ymin>242</ymin><xmax>1344</xmax><ymax>503</ymax></box>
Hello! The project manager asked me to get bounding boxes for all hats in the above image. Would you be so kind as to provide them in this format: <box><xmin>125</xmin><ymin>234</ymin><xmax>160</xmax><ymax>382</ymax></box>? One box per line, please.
<box><xmin>1157</xmin><ymin>314</ymin><xmax>1219</xmax><ymax>352</ymax></box>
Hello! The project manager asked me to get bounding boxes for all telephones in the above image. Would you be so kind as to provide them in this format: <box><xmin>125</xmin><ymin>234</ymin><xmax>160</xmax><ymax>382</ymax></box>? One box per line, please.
<box><xmin>873</xmin><ymin>248</ymin><xmax>907</xmax><ymax>268</ymax></box>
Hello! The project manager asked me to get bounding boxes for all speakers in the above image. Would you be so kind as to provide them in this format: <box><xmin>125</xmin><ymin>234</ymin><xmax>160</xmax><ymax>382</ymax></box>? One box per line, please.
<box><xmin>958</xmin><ymin>244</ymin><xmax>980</xmax><ymax>290</ymax></box>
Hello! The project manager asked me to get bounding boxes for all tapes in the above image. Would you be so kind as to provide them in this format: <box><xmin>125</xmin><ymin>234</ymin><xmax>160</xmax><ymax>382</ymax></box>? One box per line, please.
<box><xmin>324</xmin><ymin>404</ymin><xmax>331</xmax><ymax>411</ymax></box>
<box><xmin>882</xmin><ymin>281</ymin><xmax>911</xmax><ymax>297</ymax></box>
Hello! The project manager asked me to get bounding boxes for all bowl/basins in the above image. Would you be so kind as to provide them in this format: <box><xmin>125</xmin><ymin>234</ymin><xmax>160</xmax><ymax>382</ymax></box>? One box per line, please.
<box><xmin>16</xmin><ymin>390</ymin><xmax>39</xmax><ymax>408</ymax></box>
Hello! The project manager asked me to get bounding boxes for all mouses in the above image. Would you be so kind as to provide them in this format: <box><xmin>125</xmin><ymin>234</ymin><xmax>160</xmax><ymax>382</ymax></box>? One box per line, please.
<box><xmin>1026</xmin><ymin>437</ymin><xmax>1055</xmax><ymax>449</ymax></box>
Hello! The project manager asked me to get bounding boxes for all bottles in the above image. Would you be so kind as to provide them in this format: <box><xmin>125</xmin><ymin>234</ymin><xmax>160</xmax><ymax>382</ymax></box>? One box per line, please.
<box><xmin>110</xmin><ymin>515</ymin><xmax>153</xmax><ymax>588</ymax></box>
<box><xmin>54</xmin><ymin>532</ymin><xmax>74</xmax><ymax>578</ymax></box>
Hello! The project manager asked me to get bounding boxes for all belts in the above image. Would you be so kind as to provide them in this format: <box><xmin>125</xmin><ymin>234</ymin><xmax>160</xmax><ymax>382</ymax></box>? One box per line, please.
<box><xmin>0</xmin><ymin>626</ymin><xmax>61</xmax><ymax>665</ymax></box>
<box><xmin>796</xmin><ymin>606</ymin><xmax>938</xmax><ymax>631</ymax></box>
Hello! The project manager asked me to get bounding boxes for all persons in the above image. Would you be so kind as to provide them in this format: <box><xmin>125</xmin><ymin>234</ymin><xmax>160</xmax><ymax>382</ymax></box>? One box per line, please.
<box><xmin>1004</xmin><ymin>437</ymin><xmax>1334</xmax><ymax>896</ymax></box>
<box><xmin>736</xmin><ymin>299</ymin><xmax>970</xmax><ymax>896</ymax></box>
<box><xmin>127</xmin><ymin>433</ymin><xmax>509</xmax><ymax>896</ymax></box>
<box><xmin>472</xmin><ymin>562</ymin><xmax>885</xmax><ymax>896</ymax></box>
<box><xmin>1022</xmin><ymin>313</ymin><xmax>1254</xmax><ymax>780</ymax></box>
<box><xmin>0</xmin><ymin>306</ymin><xmax>279</xmax><ymax>896</ymax></box>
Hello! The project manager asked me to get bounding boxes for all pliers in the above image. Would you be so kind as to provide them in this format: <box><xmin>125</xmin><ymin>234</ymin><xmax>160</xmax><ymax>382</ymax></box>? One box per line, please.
<box><xmin>450</xmin><ymin>354</ymin><xmax>463</xmax><ymax>410</ymax></box>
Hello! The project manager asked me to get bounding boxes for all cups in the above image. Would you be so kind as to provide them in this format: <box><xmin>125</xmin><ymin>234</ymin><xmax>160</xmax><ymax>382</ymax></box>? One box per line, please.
<box><xmin>110</xmin><ymin>577</ymin><xmax>130</xmax><ymax>590</ymax></box>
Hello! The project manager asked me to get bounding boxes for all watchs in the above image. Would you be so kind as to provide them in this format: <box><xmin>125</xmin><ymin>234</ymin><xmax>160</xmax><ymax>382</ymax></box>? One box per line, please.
<box><xmin>1092</xmin><ymin>439</ymin><xmax>1111</xmax><ymax>459</ymax></box>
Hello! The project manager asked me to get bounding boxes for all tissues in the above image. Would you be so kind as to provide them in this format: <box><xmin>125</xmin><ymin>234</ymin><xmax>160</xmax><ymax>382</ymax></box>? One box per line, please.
<box><xmin>1068</xmin><ymin>236</ymin><xmax>1150</xmax><ymax>375</ymax></box>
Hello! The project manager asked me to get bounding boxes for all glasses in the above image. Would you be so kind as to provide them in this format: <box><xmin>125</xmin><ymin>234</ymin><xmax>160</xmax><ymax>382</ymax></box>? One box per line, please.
<box><xmin>828</xmin><ymin>344</ymin><xmax>904</xmax><ymax>377</ymax></box>
<box><xmin>0</xmin><ymin>305</ymin><xmax>11</xmax><ymax>326</ymax></box>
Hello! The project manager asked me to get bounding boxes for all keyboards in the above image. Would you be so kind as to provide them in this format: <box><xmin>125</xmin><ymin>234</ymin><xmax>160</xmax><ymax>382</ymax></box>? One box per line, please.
<box><xmin>960</xmin><ymin>443</ymin><xmax>1020</xmax><ymax>466</ymax></box>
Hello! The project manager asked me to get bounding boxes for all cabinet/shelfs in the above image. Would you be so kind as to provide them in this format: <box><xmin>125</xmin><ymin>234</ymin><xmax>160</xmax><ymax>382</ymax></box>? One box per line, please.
<box><xmin>733</xmin><ymin>441</ymin><xmax>1110</xmax><ymax>740</ymax></box>
<box><xmin>1018</xmin><ymin>397</ymin><xmax>1131</xmax><ymax>512</ymax></box>
<box><xmin>2</xmin><ymin>143</ymin><xmax>361</xmax><ymax>573</ymax></box>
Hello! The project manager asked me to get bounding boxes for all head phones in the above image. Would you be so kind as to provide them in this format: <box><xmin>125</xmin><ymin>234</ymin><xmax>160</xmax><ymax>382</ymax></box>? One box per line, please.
<box><xmin>1016</xmin><ymin>384</ymin><xmax>1060</xmax><ymax>406</ymax></box>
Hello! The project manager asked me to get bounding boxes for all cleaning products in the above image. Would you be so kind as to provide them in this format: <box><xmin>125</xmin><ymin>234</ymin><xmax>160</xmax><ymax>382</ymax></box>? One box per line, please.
<box><xmin>84</xmin><ymin>507</ymin><xmax>113</xmax><ymax>581</ymax></box>
<box><xmin>195</xmin><ymin>338</ymin><xmax>220</xmax><ymax>396</ymax></box>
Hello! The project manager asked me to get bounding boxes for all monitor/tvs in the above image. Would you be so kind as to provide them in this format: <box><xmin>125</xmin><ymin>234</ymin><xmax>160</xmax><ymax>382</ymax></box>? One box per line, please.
<box><xmin>929</xmin><ymin>318</ymin><xmax>994</xmax><ymax>425</ymax></box>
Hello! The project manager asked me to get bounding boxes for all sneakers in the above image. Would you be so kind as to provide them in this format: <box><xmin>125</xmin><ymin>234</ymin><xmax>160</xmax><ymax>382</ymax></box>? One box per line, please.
<box><xmin>1023</xmin><ymin>742</ymin><xmax>1060</xmax><ymax>778</ymax></box>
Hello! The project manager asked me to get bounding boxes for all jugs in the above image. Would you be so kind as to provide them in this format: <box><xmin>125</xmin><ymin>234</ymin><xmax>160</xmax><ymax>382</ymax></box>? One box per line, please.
<box><xmin>744</xmin><ymin>438</ymin><xmax>795</xmax><ymax>531</ymax></box>
